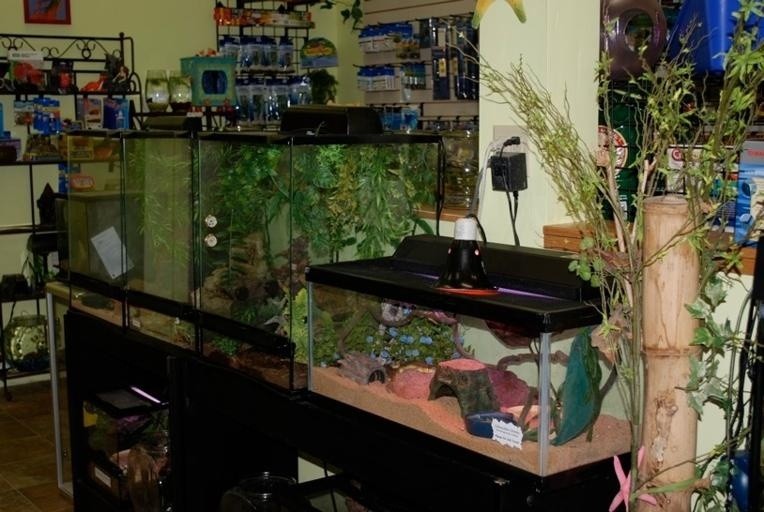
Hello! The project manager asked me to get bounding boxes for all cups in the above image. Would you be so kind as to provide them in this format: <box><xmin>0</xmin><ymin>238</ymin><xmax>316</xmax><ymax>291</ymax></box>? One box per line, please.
<box><xmin>169</xmin><ymin>73</ymin><xmax>191</xmax><ymax>112</ymax></box>
<box><xmin>221</xmin><ymin>471</ymin><xmax>297</xmax><ymax>511</ymax></box>
<box><xmin>126</xmin><ymin>431</ymin><xmax>172</xmax><ymax>511</ymax></box>
<box><xmin>145</xmin><ymin>69</ymin><xmax>168</xmax><ymax>112</ymax></box>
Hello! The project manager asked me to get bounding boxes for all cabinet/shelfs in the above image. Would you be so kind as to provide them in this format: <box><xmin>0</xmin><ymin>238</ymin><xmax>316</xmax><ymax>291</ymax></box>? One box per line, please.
<box><xmin>214</xmin><ymin>1</ymin><xmax>316</xmax><ymax>78</ymax></box>
<box><xmin>0</xmin><ymin>32</ymin><xmax>144</xmax><ymax>398</ymax></box>
<box><xmin>352</xmin><ymin>11</ymin><xmax>478</xmax><ymax>130</ymax></box>
<box><xmin>63</xmin><ymin>107</ymin><xmax>446</xmax><ymax>512</ymax></box>
<box><xmin>304</xmin><ymin>235</ymin><xmax>642</xmax><ymax>512</ymax></box>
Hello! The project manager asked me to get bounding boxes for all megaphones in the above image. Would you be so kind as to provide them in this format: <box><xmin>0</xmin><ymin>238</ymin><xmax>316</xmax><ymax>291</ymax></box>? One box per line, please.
<box><xmin>428</xmin><ymin>217</ymin><xmax>499</xmax><ymax>295</ymax></box>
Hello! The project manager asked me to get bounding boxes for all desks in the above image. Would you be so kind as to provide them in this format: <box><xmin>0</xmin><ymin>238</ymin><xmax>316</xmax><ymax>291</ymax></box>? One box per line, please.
<box><xmin>543</xmin><ymin>219</ymin><xmax>756</xmax><ymax>277</ymax></box>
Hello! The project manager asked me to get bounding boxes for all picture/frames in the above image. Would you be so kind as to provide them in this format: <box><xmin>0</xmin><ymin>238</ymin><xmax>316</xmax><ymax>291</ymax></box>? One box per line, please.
<box><xmin>23</xmin><ymin>0</ymin><xmax>71</xmax><ymax>25</ymax></box>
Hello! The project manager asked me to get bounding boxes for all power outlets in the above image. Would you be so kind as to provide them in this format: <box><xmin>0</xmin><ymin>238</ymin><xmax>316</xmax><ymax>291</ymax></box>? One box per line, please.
<box><xmin>493</xmin><ymin>125</ymin><xmax>528</xmax><ymax>155</ymax></box>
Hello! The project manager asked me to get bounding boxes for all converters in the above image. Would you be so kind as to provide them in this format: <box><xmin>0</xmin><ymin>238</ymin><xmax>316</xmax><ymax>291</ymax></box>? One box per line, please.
<box><xmin>492</xmin><ymin>152</ymin><xmax>527</xmax><ymax>192</ymax></box>
